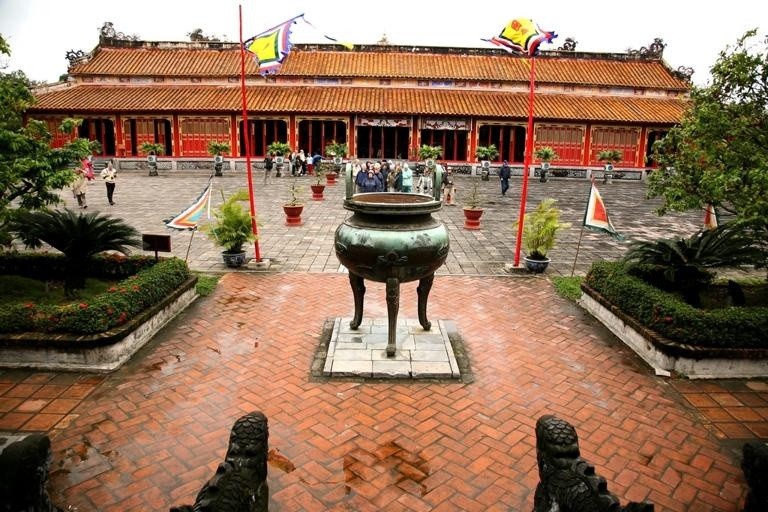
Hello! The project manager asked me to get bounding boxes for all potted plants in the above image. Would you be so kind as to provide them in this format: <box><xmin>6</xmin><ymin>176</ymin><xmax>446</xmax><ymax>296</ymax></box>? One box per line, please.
<box><xmin>462</xmin><ymin>174</ymin><xmax>486</xmax><ymax>230</ymax></box>
<box><xmin>325</xmin><ymin>161</ymin><xmax>339</xmax><ymax>184</ymax></box>
<box><xmin>324</xmin><ymin>140</ymin><xmax>348</xmax><ymax>164</ymax></box>
<box><xmin>266</xmin><ymin>141</ymin><xmax>292</xmax><ymax>164</ymax></box>
<box><xmin>281</xmin><ymin>174</ymin><xmax>305</xmax><ymax>227</ymax></box>
<box><xmin>199</xmin><ymin>187</ymin><xmax>262</xmax><ymax>268</ymax></box>
<box><xmin>310</xmin><ymin>168</ymin><xmax>327</xmax><ymax>201</ymax></box>
<box><xmin>417</xmin><ymin>144</ymin><xmax>445</xmax><ymax>167</ymax></box>
<box><xmin>139</xmin><ymin>140</ymin><xmax>165</xmax><ymax>162</ymax></box>
<box><xmin>533</xmin><ymin>144</ymin><xmax>559</xmax><ymax>170</ymax></box>
<box><xmin>508</xmin><ymin>197</ymin><xmax>574</xmax><ymax>274</ymax></box>
<box><xmin>206</xmin><ymin>140</ymin><xmax>231</xmax><ymax>163</ymax></box>
<box><xmin>596</xmin><ymin>149</ymin><xmax>624</xmax><ymax>171</ymax></box>
<box><xmin>475</xmin><ymin>144</ymin><xmax>500</xmax><ymax>168</ymax></box>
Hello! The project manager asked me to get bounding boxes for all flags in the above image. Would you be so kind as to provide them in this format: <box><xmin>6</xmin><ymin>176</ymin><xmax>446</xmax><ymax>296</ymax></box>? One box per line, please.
<box><xmin>488</xmin><ymin>18</ymin><xmax>558</xmax><ymax>59</ymax></box>
<box><xmin>242</xmin><ymin>20</ymin><xmax>294</xmax><ymax>77</ymax></box>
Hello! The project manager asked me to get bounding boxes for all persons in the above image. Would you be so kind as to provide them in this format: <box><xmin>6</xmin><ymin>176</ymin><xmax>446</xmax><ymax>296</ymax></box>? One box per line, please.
<box><xmin>263</xmin><ymin>148</ymin><xmax>325</xmax><ymax>185</ymax></box>
<box><xmin>499</xmin><ymin>160</ymin><xmax>511</xmax><ymax>196</ymax></box>
<box><xmin>100</xmin><ymin>162</ymin><xmax>117</xmax><ymax>205</ymax></box>
<box><xmin>70</xmin><ymin>167</ymin><xmax>88</xmax><ymax>209</ymax></box>
<box><xmin>352</xmin><ymin>159</ymin><xmax>455</xmax><ymax>206</ymax></box>
